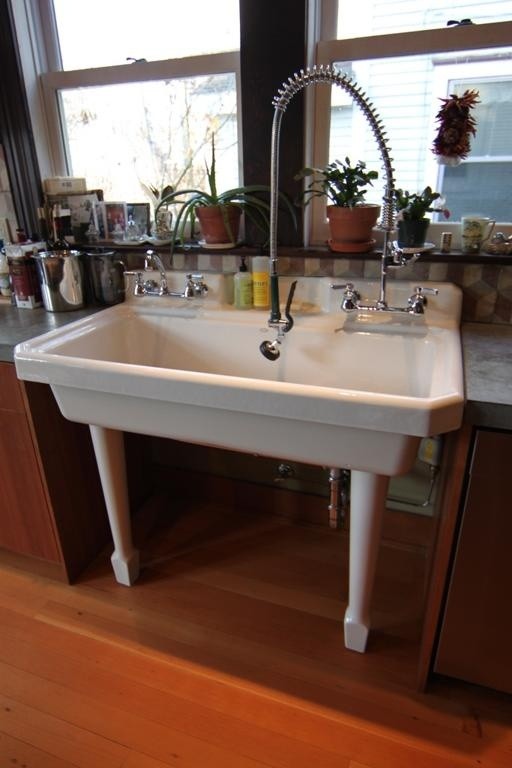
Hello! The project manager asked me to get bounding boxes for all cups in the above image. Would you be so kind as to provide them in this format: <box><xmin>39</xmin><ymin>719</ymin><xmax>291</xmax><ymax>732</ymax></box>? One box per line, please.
<box><xmin>84</xmin><ymin>249</ymin><xmax>129</xmax><ymax>306</ymax></box>
<box><xmin>461</xmin><ymin>215</ymin><xmax>496</xmax><ymax>253</ymax></box>
<box><xmin>0</xmin><ymin>272</ymin><xmax>17</xmax><ymax>297</ymax></box>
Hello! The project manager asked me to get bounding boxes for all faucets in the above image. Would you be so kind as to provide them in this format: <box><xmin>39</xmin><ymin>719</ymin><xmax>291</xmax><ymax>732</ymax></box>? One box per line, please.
<box><xmin>258</xmin><ymin>67</ymin><xmax>394</xmax><ymax>362</ymax></box>
<box><xmin>142</xmin><ymin>250</ymin><xmax>169</xmax><ymax>296</ymax></box>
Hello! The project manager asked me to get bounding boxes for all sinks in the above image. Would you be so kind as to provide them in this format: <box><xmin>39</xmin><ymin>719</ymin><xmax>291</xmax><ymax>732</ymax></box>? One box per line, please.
<box><xmin>13</xmin><ymin>304</ymin><xmax>463</xmax><ymax>476</ymax></box>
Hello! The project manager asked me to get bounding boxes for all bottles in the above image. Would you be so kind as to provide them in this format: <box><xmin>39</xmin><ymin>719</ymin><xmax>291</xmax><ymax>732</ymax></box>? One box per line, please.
<box><xmin>252</xmin><ymin>255</ymin><xmax>272</xmax><ymax>310</ymax></box>
<box><xmin>85</xmin><ymin>219</ymin><xmax>148</xmax><ymax>246</ymax></box>
<box><xmin>440</xmin><ymin>232</ymin><xmax>453</xmax><ymax>253</ymax></box>
<box><xmin>15</xmin><ymin>227</ymin><xmax>30</xmax><ymax>243</ymax></box>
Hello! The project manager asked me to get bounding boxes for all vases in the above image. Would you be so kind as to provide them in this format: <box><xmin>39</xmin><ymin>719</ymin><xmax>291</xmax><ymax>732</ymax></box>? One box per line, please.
<box><xmin>435</xmin><ymin>154</ymin><xmax>462</xmax><ymax>168</ymax></box>
<box><xmin>396</xmin><ymin>215</ymin><xmax>429</xmax><ymax>248</ymax></box>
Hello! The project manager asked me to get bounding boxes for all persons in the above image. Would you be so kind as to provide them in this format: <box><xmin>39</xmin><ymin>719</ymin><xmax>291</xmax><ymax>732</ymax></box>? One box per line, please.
<box><xmin>117</xmin><ymin>212</ymin><xmax>124</xmax><ymax>230</ymax></box>
<box><xmin>107</xmin><ymin>211</ymin><xmax>113</xmax><ymax>232</ymax></box>
<box><xmin>112</xmin><ymin>217</ymin><xmax>122</xmax><ymax>231</ymax></box>
<box><xmin>127</xmin><ymin>211</ymin><xmax>139</xmax><ymax>232</ymax></box>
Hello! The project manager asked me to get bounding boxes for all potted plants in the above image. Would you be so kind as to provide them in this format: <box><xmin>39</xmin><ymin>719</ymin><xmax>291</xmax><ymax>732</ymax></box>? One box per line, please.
<box><xmin>294</xmin><ymin>155</ymin><xmax>383</xmax><ymax>253</ymax></box>
<box><xmin>138</xmin><ymin>176</ymin><xmax>179</xmax><ymax>243</ymax></box>
<box><xmin>151</xmin><ymin>127</ymin><xmax>286</xmax><ymax>249</ymax></box>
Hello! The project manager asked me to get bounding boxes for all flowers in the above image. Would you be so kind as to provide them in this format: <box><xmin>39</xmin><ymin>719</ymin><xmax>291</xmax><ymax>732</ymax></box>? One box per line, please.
<box><xmin>426</xmin><ymin>86</ymin><xmax>482</xmax><ymax>156</ymax></box>
<box><xmin>381</xmin><ymin>186</ymin><xmax>456</xmax><ymax>221</ymax></box>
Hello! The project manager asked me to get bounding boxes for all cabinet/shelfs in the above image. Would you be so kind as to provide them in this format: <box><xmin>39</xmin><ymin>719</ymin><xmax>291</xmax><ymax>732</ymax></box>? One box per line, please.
<box><xmin>1</xmin><ymin>287</ymin><xmax>171</xmax><ymax>589</ymax></box>
<box><xmin>407</xmin><ymin>319</ymin><xmax>511</xmax><ymax>705</ymax></box>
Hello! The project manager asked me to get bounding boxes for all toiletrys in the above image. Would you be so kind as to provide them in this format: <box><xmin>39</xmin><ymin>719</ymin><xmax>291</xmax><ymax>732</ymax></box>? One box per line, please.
<box><xmin>253</xmin><ymin>256</ymin><xmax>270</xmax><ymax>310</ymax></box>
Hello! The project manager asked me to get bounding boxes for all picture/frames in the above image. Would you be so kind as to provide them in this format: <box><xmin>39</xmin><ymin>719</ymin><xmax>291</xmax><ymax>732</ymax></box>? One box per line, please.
<box><xmin>100</xmin><ymin>200</ymin><xmax>128</xmax><ymax>240</ymax></box>
<box><xmin>126</xmin><ymin>202</ymin><xmax>151</xmax><ymax>237</ymax></box>
